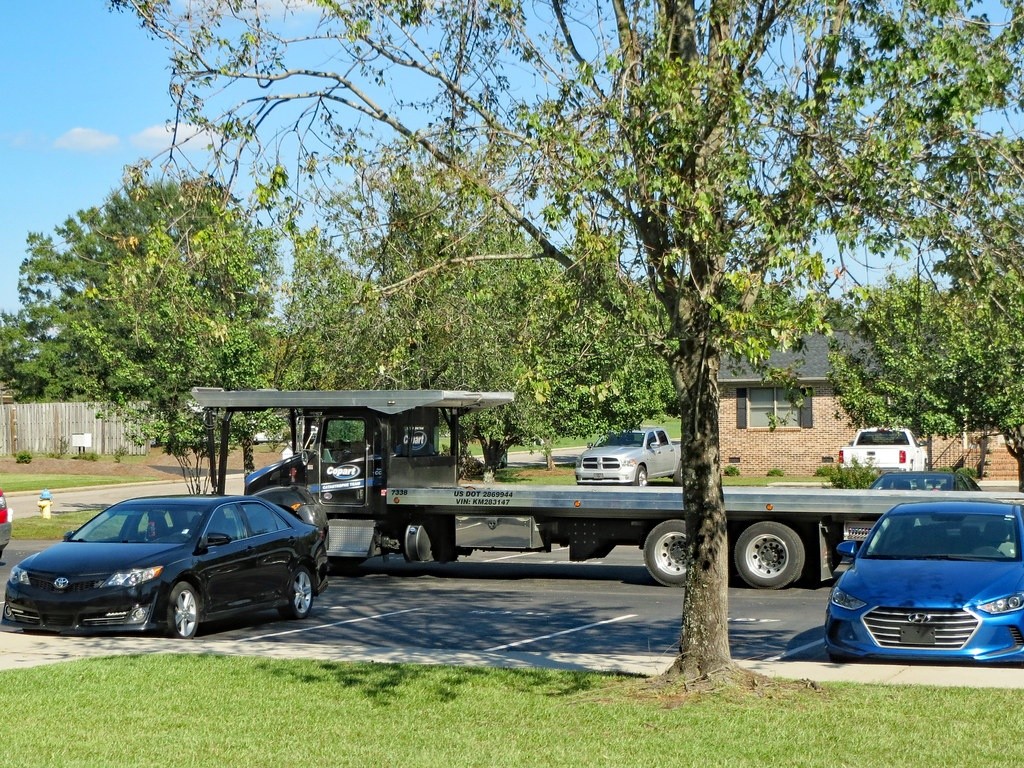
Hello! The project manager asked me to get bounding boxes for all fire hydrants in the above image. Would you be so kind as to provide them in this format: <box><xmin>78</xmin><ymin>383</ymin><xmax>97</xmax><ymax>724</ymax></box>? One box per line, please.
<box><xmin>37</xmin><ymin>488</ymin><xmax>53</xmax><ymax>520</ymax></box>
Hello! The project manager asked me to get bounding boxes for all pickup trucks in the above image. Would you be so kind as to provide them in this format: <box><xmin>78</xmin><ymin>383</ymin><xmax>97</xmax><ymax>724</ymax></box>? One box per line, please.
<box><xmin>574</xmin><ymin>427</ymin><xmax>683</xmax><ymax>487</ymax></box>
<box><xmin>838</xmin><ymin>427</ymin><xmax>928</xmax><ymax>473</ymax></box>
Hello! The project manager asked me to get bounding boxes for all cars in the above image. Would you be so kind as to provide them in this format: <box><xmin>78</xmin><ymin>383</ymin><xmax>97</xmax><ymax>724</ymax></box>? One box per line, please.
<box><xmin>252</xmin><ymin>429</ymin><xmax>283</xmax><ymax>445</ymax></box>
<box><xmin>0</xmin><ymin>494</ymin><xmax>329</xmax><ymax>639</ymax></box>
<box><xmin>823</xmin><ymin>498</ymin><xmax>1024</xmax><ymax>665</ymax></box>
<box><xmin>868</xmin><ymin>472</ymin><xmax>981</xmax><ymax>491</ymax></box>
<box><xmin>0</xmin><ymin>488</ymin><xmax>12</xmax><ymax>558</ymax></box>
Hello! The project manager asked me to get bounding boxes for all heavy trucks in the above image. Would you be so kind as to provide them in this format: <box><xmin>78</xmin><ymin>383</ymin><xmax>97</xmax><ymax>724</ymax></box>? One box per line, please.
<box><xmin>191</xmin><ymin>387</ymin><xmax>1024</xmax><ymax>592</ymax></box>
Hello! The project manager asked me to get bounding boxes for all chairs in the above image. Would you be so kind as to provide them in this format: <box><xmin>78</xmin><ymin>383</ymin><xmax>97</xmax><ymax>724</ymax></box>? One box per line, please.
<box><xmin>982</xmin><ymin>521</ymin><xmax>1009</xmax><ymax>548</ymax></box>
<box><xmin>896</xmin><ymin>481</ymin><xmax>911</xmax><ymax>490</ymax></box>
<box><xmin>862</xmin><ymin>436</ymin><xmax>873</xmax><ymax>445</ymax></box>
